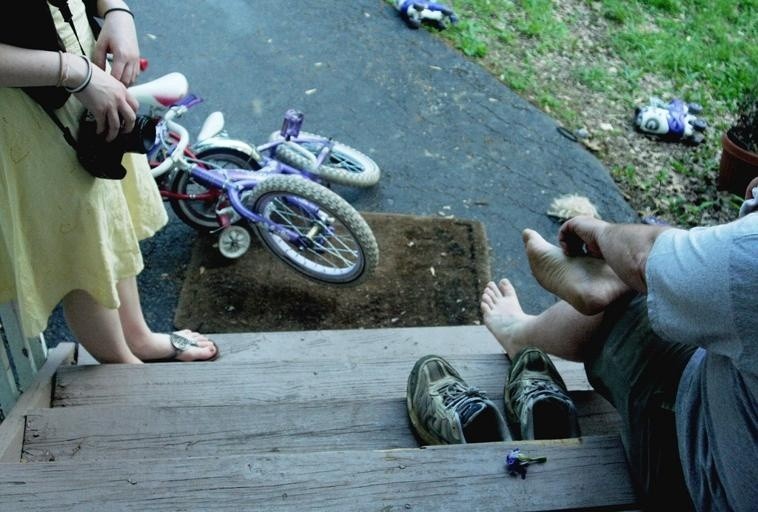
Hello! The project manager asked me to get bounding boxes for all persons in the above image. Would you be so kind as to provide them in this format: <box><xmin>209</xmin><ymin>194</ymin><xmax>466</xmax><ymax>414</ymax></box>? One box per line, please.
<box><xmin>476</xmin><ymin>180</ymin><xmax>758</xmax><ymax>512</ymax></box>
<box><xmin>1</xmin><ymin>1</ymin><xmax>221</xmax><ymax>367</ymax></box>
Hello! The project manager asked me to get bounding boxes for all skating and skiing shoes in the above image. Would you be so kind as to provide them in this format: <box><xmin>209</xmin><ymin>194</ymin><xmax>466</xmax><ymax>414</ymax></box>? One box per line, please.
<box><xmin>635</xmin><ymin>95</ymin><xmax>706</xmax><ymax>145</ymax></box>
<box><xmin>396</xmin><ymin>0</ymin><xmax>458</xmax><ymax>33</ymax></box>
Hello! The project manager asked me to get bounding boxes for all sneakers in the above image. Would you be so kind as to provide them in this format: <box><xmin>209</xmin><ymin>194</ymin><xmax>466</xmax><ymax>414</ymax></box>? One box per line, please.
<box><xmin>407</xmin><ymin>354</ymin><xmax>512</xmax><ymax>447</ymax></box>
<box><xmin>503</xmin><ymin>345</ymin><xmax>583</xmax><ymax>440</ymax></box>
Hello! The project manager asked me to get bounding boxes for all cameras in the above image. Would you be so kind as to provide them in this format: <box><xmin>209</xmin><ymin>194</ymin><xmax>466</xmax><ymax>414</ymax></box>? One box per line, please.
<box><xmin>76</xmin><ymin>106</ymin><xmax>156</xmax><ymax>180</ymax></box>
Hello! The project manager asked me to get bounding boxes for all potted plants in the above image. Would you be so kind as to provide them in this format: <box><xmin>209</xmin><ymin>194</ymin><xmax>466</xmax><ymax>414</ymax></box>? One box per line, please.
<box><xmin>716</xmin><ymin>87</ymin><xmax>758</xmax><ymax>200</ymax></box>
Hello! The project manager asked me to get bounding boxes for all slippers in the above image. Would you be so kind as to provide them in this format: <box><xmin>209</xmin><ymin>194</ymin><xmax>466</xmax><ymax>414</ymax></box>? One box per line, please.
<box><xmin>143</xmin><ymin>327</ymin><xmax>221</xmax><ymax>363</ymax></box>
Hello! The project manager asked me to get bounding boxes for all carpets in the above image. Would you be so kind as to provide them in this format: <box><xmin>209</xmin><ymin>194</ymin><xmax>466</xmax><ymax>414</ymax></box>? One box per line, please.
<box><xmin>171</xmin><ymin>211</ymin><xmax>491</xmax><ymax>336</ymax></box>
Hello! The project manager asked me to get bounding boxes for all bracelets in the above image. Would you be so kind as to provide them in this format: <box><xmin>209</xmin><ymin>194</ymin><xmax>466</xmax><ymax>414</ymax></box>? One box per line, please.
<box><xmin>55</xmin><ymin>49</ymin><xmax>64</xmax><ymax>88</ymax></box>
<box><xmin>103</xmin><ymin>8</ymin><xmax>135</xmax><ymax>20</ymax></box>
<box><xmin>65</xmin><ymin>56</ymin><xmax>93</xmax><ymax>95</ymax></box>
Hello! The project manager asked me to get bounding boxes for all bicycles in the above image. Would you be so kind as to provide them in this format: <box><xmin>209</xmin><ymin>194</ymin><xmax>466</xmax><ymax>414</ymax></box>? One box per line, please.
<box><xmin>146</xmin><ymin>93</ymin><xmax>381</xmax><ymax>287</ymax></box>
<box><xmin>124</xmin><ymin>71</ymin><xmax>260</xmax><ymax>233</ymax></box>
